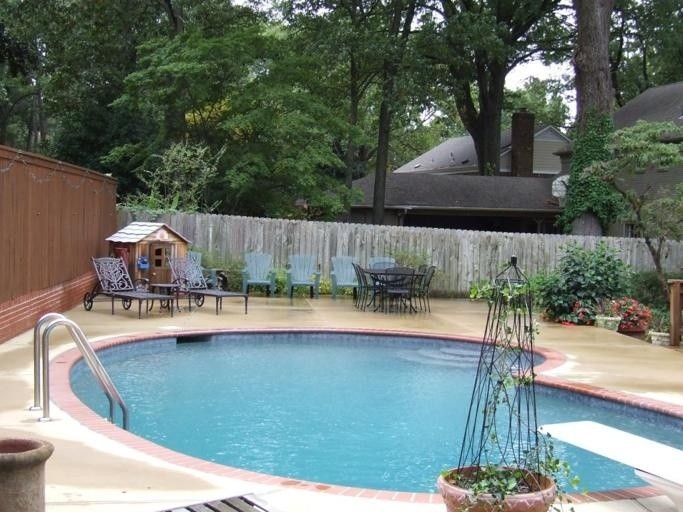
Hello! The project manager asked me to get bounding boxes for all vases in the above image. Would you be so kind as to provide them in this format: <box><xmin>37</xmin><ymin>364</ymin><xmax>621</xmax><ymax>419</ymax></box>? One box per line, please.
<box><xmin>594</xmin><ymin>315</ymin><xmax>621</xmax><ymax>332</ymax></box>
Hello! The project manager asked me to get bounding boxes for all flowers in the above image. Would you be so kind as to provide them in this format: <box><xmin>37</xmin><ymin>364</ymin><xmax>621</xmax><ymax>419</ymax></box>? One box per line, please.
<box><xmin>562</xmin><ymin>296</ymin><xmax>653</xmax><ymax>330</ymax></box>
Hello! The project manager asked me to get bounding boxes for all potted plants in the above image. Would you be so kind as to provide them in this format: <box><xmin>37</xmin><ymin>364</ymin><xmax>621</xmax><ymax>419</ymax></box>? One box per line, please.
<box><xmin>646</xmin><ymin>309</ymin><xmax>672</xmax><ymax>345</ymax></box>
<box><xmin>436</xmin><ymin>258</ymin><xmax>578</xmax><ymax>512</ymax></box>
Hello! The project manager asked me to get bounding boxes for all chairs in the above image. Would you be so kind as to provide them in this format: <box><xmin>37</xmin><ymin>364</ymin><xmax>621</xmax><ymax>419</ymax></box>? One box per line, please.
<box><xmin>242</xmin><ymin>249</ymin><xmax>396</xmax><ymax>300</ymax></box>
<box><xmin>84</xmin><ymin>255</ymin><xmax>249</xmax><ymax>321</ymax></box>
<box><xmin>351</xmin><ymin>259</ymin><xmax>437</xmax><ymax>316</ymax></box>
